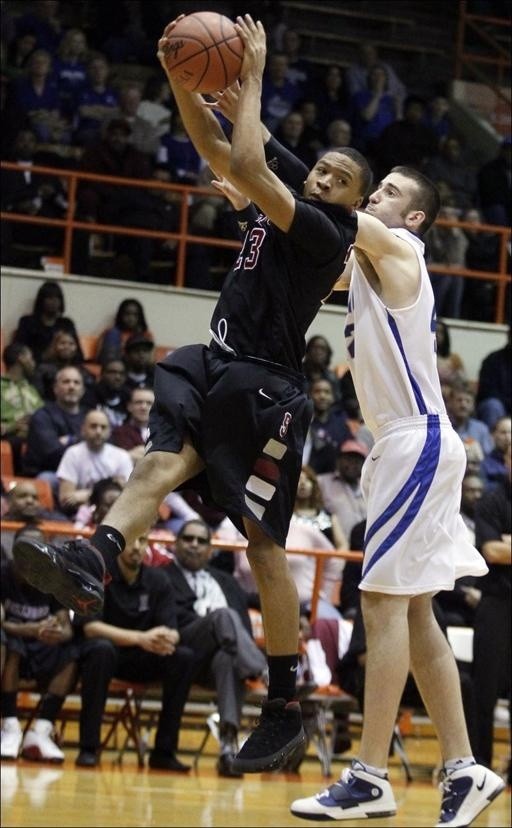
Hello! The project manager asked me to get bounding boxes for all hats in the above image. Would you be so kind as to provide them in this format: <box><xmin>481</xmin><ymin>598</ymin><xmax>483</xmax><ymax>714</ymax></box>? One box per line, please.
<box><xmin>340</xmin><ymin>438</ymin><xmax>369</xmax><ymax>458</ymax></box>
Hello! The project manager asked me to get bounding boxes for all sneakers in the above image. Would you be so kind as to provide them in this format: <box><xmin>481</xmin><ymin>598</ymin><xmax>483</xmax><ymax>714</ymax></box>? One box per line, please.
<box><xmin>0</xmin><ymin>728</ymin><xmax>24</xmax><ymax>758</ymax></box>
<box><xmin>290</xmin><ymin>766</ymin><xmax>397</xmax><ymax>820</ymax></box>
<box><xmin>236</xmin><ymin>697</ymin><xmax>306</xmax><ymax>769</ymax></box>
<box><xmin>12</xmin><ymin>527</ymin><xmax>107</xmax><ymax>618</ymax></box>
<box><xmin>436</xmin><ymin>762</ymin><xmax>507</xmax><ymax>827</ymax></box>
<box><xmin>21</xmin><ymin>729</ymin><xmax>65</xmax><ymax>761</ymax></box>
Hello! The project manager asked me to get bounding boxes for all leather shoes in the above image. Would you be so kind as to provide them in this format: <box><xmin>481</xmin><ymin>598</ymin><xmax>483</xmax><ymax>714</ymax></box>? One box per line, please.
<box><xmin>149</xmin><ymin>748</ymin><xmax>191</xmax><ymax>770</ymax></box>
<box><xmin>220</xmin><ymin>752</ymin><xmax>243</xmax><ymax>775</ymax></box>
<box><xmin>75</xmin><ymin>750</ymin><xmax>98</xmax><ymax>765</ymax></box>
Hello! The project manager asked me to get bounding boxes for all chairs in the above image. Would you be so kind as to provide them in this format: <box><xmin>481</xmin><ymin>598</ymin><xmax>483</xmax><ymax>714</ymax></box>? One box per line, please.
<box><xmin>0</xmin><ymin>326</ymin><xmax>415</xmax><ymax>792</ymax></box>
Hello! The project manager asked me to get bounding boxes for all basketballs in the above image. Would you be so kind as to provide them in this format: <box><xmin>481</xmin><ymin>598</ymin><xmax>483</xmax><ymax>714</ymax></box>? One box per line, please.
<box><xmin>164</xmin><ymin>11</ymin><xmax>244</xmax><ymax>93</ymax></box>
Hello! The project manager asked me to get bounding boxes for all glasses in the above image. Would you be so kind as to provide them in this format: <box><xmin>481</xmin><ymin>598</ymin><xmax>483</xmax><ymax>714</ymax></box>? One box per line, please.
<box><xmin>179</xmin><ymin>532</ymin><xmax>208</xmax><ymax>543</ymax></box>
